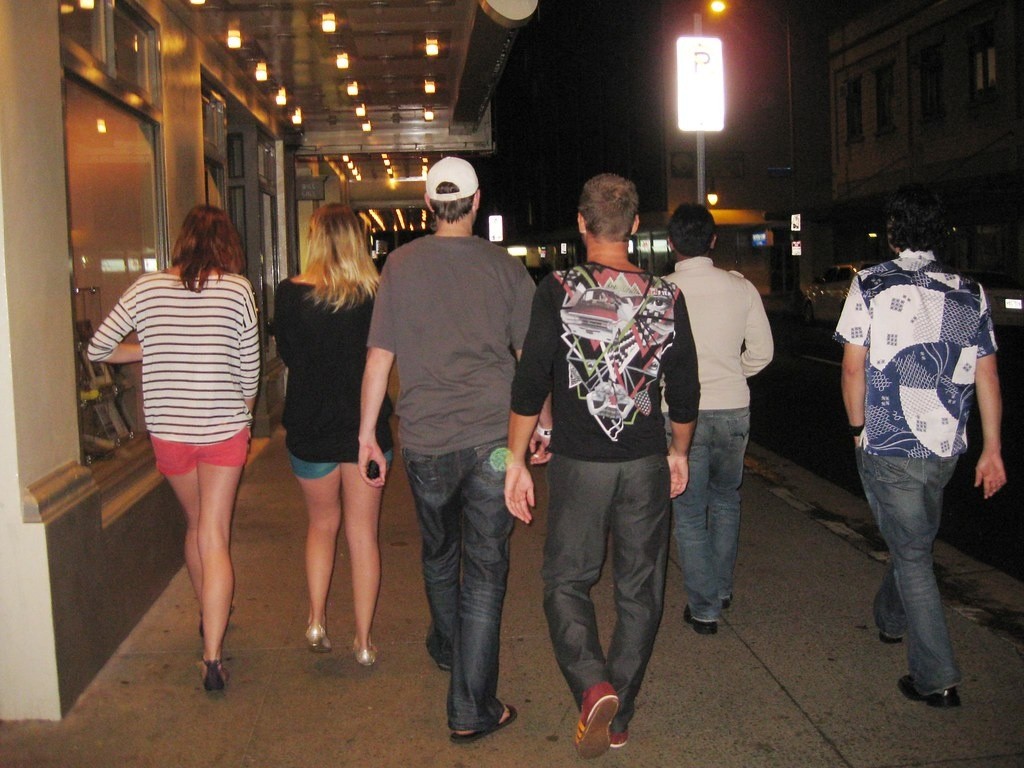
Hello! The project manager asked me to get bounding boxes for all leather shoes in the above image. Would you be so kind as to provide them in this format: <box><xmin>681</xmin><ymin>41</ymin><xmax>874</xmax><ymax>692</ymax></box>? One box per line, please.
<box><xmin>878</xmin><ymin>632</ymin><xmax>903</xmax><ymax>645</ymax></box>
<box><xmin>898</xmin><ymin>674</ymin><xmax>960</xmax><ymax>709</ymax></box>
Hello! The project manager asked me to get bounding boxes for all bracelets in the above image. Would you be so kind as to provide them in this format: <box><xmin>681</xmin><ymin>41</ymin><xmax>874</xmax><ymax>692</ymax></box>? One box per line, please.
<box><xmin>536</xmin><ymin>425</ymin><xmax>553</xmax><ymax>438</ymax></box>
<box><xmin>848</xmin><ymin>425</ymin><xmax>864</xmax><ymax>437</ymax></box>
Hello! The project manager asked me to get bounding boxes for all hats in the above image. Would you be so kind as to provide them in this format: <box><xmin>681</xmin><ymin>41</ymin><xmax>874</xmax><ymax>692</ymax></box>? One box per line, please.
<box><xmin>426</xmin><ymin>156</ymin><xmax>479</xmax><ymax>201</ymax></box>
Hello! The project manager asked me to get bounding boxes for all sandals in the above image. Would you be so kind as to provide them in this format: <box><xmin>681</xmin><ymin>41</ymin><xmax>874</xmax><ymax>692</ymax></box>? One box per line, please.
<box><xmin>437</xmin><ymin>657</ymin><xmax>451</xmax><ymax>672</ymax></box>
<box><xmin>202</xmin><ymin>656</ymin><xmax>231</xmax><ymax>691</ymax></box>
<box><xmin>451</xmin><ymin>704</ymin><xmax>517</xmax><ymax>745</ymax></box>
<box><xmin>198</xmin><ymin>605</ymin><xmax>235</xmax><ymax>638</ymax></box>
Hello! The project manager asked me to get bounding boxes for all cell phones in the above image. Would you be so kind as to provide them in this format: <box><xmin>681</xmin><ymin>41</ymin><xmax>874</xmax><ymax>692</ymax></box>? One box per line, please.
<box><xmin>367</xmin><ymin>461</ymin><xmax>380</xmax><ymax>481</ymax></box>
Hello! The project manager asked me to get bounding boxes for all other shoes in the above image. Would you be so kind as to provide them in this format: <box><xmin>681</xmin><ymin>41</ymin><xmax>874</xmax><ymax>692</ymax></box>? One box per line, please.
<box><xmin>353</xmin><ymin>637</ymin><xmax>376</xmax><ymax>667</ymax></box>
<box><xmin>683</xmin><ymin>604</ymin><xmax>718</xmax><ymax>635</ymax></box>
<box><xmin>304</xmin><ymin>624</ymin><xmax>332</xmax><ymax>653</ymax></box>
<box><xmin>720</xmin><ymin>594</ymin><xmax>734</xmax><ymax>610</ymax></box>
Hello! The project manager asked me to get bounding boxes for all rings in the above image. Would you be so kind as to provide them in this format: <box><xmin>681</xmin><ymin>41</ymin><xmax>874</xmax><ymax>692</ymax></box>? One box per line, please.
<box><xmin>533</xmin><ymin>454</ymin><xmax>538</xmax><ymax>458</ymax></box>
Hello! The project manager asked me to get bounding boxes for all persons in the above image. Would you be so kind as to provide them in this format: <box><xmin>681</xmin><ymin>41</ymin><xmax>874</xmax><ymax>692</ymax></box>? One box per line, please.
<box><xmin>832</xmin><ymin>192</ymin><xmax>1008</xmax><ymax>706</ymax></box>
<box><xmin>659</xmin><ymin>205</ymin><xmax>774</xmax><ymax>631</ymax></box>
<box><xmin>359</xmin><ymin>157</ymin><xmax>555</xmax><ymax>745</ymax></box>
<box><xmin>270</xmin><ymin>204</ymin><xmax>396</xmax><ymax>663</ymax></box>
<box><xmin>87</xmin><ymin>203</ymin><xmax>261</xmax><ymax>692</ymax></box>
<box><xmin>503</xmin><ymin>173</ymin><xmax>700</xmax><ymax>761</ymax></box>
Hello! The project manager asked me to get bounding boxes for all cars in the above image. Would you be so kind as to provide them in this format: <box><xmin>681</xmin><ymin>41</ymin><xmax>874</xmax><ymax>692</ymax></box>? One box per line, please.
<box><xmin>953</xmin><ymin>267</ymin><xmax>1024</xmax><ymax>326</ymax></box>
<box><xmin>796</xmin><ymin>259</ymin><xmax>880</xmax><ymax>325</ymax></box>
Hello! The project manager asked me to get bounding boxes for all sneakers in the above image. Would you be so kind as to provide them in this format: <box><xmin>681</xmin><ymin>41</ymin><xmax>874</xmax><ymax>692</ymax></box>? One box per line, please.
<box><xmin>575</xmin><ymin>681</ymin><xmax>619</xmax><ymax>760</ymax></box>
<box><xmin>608</xmin><ymin>726</ymin><xmax>628</xmax><ymax>750</ymax></box>
